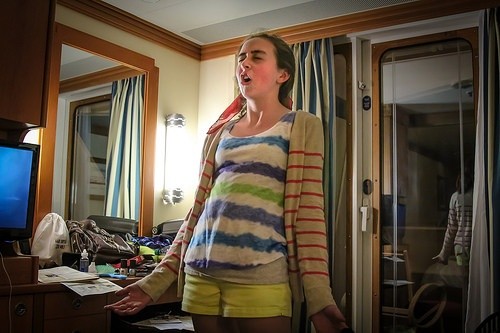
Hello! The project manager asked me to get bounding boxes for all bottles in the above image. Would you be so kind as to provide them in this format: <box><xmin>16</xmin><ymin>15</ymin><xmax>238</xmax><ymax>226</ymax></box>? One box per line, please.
<box><xmin>129</xmin><ymin>260</ymin><xmax>136</xmax><ymax>277</ymax></box>
<box><xmin>120</xmin><ymin>259</ymin><xmax>127</xmax><ymax>275</ymax></box>
<box><xmin>80</xmin><ymin>248</ymin><xmax>89</xmax><ymax>272</ymax></box>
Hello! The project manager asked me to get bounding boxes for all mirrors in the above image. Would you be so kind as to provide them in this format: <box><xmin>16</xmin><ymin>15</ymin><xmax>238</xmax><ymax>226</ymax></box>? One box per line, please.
<box><xmin>33</xmin><ymin>21</ymin><xmax>160</xmax><ymax>238</ymax></box>
<box><xmin>372</xmin><ymin>26</ymin><xmax>481</xmax><ymax>333</ymax></box>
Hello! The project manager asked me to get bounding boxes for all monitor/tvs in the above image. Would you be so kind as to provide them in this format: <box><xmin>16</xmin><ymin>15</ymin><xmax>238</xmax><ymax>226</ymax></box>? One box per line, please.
<box><xmin>0</xmin><ymin>142</ymin><xmax>41</xmax><ymax>240</ymax></box>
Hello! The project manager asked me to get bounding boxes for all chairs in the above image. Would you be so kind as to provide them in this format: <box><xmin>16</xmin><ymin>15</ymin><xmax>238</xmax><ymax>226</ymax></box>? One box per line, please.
<box><xmin>383</xmin><ymin>250</ymin><xmax>447</xmax><ymax>333</ymax></box>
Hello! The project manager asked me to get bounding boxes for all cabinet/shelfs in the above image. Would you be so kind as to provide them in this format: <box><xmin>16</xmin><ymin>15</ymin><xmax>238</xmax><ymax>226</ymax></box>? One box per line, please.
<box><xmin>0</xmin><ymin>0</ymin><xmax>56</xmax><ymax>129</ymax></box>
<box><xmin>0</xmin><ymin>279</ymin><xmax>182</xmax><ymax>333</ymax></box>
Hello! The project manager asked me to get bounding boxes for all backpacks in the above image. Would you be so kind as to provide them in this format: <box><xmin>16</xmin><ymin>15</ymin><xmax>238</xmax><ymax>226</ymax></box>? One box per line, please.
<box><xmin>66</xmin><ymin>218</ymin><xmax>135</xmax><ymax>265</ymax></box>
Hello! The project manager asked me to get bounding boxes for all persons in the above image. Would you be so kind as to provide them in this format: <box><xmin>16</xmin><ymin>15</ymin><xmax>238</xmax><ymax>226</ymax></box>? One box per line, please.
<box><xmin>104</xmin><ymin>35</ymin><xmax>348</xmax><ymax>333</ymax></box>
<box><xmin>431</xmin><ymin>169</ymin><xmax>474</xmax><ymax>333</ymax></box>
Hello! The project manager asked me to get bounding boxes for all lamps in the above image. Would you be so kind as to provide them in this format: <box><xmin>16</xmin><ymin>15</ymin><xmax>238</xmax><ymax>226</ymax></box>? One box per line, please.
<box><xmin>162</xmin><ymin>111</ymin><xmax>188</xmax><ymax>205</ymax></box>
